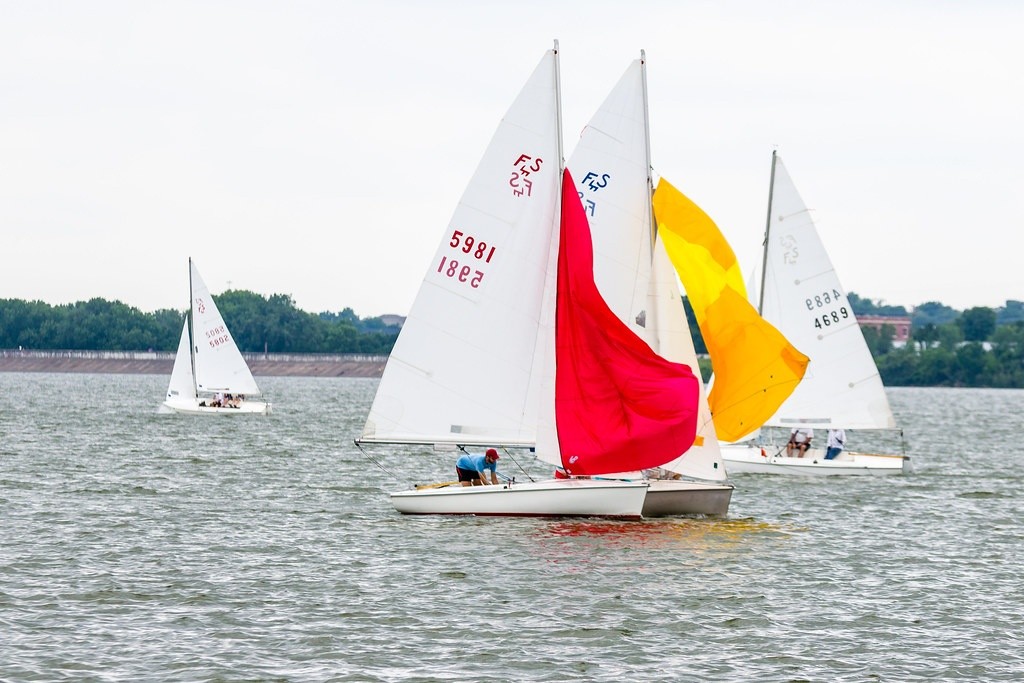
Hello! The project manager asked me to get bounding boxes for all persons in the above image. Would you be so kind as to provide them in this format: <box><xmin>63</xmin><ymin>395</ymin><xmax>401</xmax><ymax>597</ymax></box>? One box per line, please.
<box><xmin>787</xmin><ymin>428</ymin><xmax>815</xmax><ymax>458</ymax></box>
<box><xmin>213</xmin><ymin>392</ymin><xmax>244</xmax><ymax>409</ymax></box>
<box><xmin>456</xmin><ymin>449</ymin><xmax>499</xmax><ymax>486</ymax></box>
<box><xmin>824</xmin><ymin>429</ymin><xmax>847</xmax><ymax>460</ymax></box>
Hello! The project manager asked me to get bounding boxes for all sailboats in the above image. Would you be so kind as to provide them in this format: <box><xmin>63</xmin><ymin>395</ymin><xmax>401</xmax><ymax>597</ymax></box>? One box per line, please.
<box><xmin>158</xmin><ymin>255</ymin><xmax>270</xmax><ymax>416</ymax></box>
<box><xmin>347</xmin><ymin>36</ymin><xmax>703</xmax><ymax>522</ymax></box>
<box><xmin>573</xmin><ymin>46</ymin><xmax>812</xmax><ymax>520</ymax></box>
<box><xmin>694</xmin><ymin>150</ymin><xmax>914</xmax><ymax>477</ymax></box>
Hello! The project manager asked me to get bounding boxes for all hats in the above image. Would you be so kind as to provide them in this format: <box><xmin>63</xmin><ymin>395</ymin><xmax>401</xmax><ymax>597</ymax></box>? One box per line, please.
<box><xmin>486</xmin><ymin>449</ymin><xmax>499</xmax><ymax>459</ymax></box>
